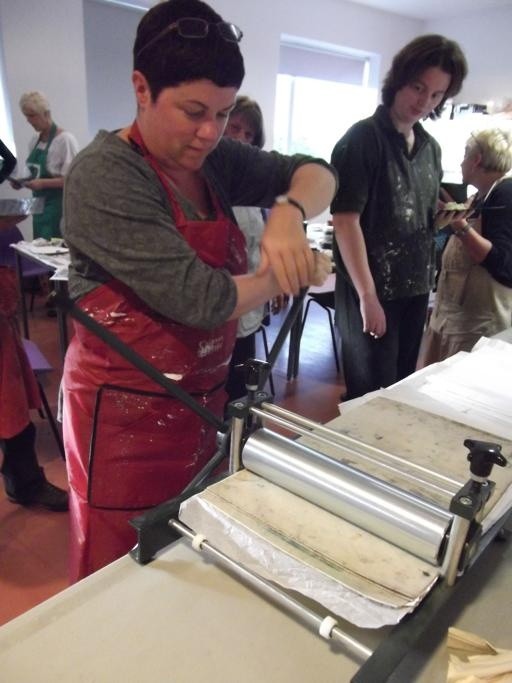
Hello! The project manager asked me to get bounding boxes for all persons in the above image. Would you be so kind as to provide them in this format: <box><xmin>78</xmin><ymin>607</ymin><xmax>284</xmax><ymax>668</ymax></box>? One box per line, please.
<box><xmin>328</xmin><ymin>31</ymin><xmax>473</xmax><ymax>397</ymax></box>
<box><xmin>8</xmin><ymin>90</ymin><xmax>76</xmax><ymax>239</ymax></box>
<box><xmin>56</xmin><ymin>0</ymin><xmax>341</xmax><ymax>592</ymax></box>
<box><xmin>412</xmin><ymin>128</ymin><xmax>512</xmax><ymax>374</ymax></box>
<box><xmin>0</xmin><ymin>264</ymin><xmax>70</xmax><ymax>513</ymax></box>
<box><xmin>219</xmin><ymin>91</ymin><xmax>269</xmax><ymax>452</ymax></box>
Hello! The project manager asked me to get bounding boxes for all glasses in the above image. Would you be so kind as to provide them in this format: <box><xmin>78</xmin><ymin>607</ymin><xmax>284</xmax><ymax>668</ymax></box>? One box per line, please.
<box><xmin>137</xmin><ymin>17</ymin><xmax>244</xmax><ymax>55</ymax></box>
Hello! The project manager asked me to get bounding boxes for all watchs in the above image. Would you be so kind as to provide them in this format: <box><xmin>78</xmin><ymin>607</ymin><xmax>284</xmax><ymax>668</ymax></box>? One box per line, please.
<box><xmin>273</xmin><ymin>194</ymin><xmax>307</xmax><ymax>220</ymax></box>
<box><xmin>454</xmin><ymin>223</ymin><xmax>472</xmax><ymax>239</ymax></box>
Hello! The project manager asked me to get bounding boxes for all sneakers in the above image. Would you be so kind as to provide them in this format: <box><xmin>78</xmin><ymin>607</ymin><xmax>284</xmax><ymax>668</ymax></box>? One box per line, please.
<box><xmin>5</xmin><ymin>479</ymin><xmax>68</xmax><ymax>511</ymax></box>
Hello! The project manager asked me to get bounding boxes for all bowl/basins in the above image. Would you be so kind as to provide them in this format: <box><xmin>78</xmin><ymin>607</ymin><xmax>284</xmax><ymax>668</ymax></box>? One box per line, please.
<box><xmin>438</xmin><ymin>209</ymin><xmax>475</xmax><ymax>219</ymax></box>
